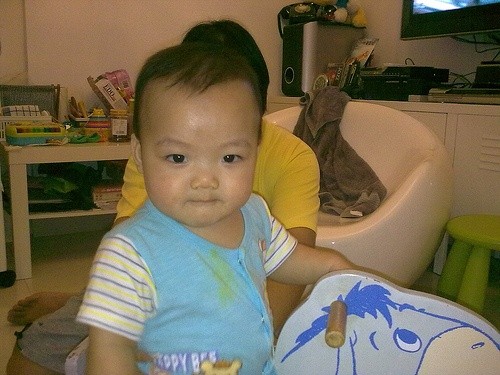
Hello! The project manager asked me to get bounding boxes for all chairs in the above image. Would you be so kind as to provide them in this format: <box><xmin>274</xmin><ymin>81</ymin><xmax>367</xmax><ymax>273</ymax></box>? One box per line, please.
<box><xmin>263</xmin><ymin>101</ymin><xmax>456</xmax><ymax>288</ymax></box>
<box><xmin>0</xmin><ymin>83</ymin><xmax>60</xmax><ymax>121</ymax></box>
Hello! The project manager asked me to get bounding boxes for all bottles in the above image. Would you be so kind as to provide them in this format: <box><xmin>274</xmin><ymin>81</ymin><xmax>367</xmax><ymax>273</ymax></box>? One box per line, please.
<box><xmin>108</xmin><ymin>109</ymin><xmax>131</xmax><ymax>142</ymax></box>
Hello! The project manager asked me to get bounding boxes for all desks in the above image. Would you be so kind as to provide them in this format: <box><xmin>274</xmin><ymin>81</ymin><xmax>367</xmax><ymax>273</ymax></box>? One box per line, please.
<box><xmin>1</xmin><ymin>141</ymin><xmax>131</xmax><ymax>281</ymax></box>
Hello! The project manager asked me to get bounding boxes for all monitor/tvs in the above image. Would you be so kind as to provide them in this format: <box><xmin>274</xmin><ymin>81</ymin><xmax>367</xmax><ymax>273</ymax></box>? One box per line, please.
<box><xmin>400</xmin><ymin>0</ymin><xmax>500</xmax><ymax>40</ymax></box>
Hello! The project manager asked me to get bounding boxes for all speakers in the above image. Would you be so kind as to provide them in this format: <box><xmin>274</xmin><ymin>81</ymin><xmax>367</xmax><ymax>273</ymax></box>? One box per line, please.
<box><xmin>281</xmin><ymin>21</ymin><xmax>363</xmax><ymax>96</ymax></box>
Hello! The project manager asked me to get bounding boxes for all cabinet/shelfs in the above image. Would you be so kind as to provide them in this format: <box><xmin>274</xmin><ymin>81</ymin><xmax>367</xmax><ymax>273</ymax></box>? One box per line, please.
<box><xmin>267</xmin><ymin>96</ymin><xmax>500</xmax><ymax>275</ymax></box>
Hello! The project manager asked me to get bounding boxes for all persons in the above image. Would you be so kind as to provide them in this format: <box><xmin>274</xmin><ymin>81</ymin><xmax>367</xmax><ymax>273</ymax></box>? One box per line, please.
<box><xmin>76</xmin><ymin>44</ymin><xmax>356</xmax><ymax>375</ymax></box>
<box><xmin>6</xmin><ymin>20</ymin><xmax>320</xmax><ymax>375</ymax></box>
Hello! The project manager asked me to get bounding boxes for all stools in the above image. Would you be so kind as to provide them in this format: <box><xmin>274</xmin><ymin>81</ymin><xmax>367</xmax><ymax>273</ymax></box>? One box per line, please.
<box><xmin>437</xmin><ymin>214</ymin><xmax>500</xmax><ymax>311</ymax></box>
